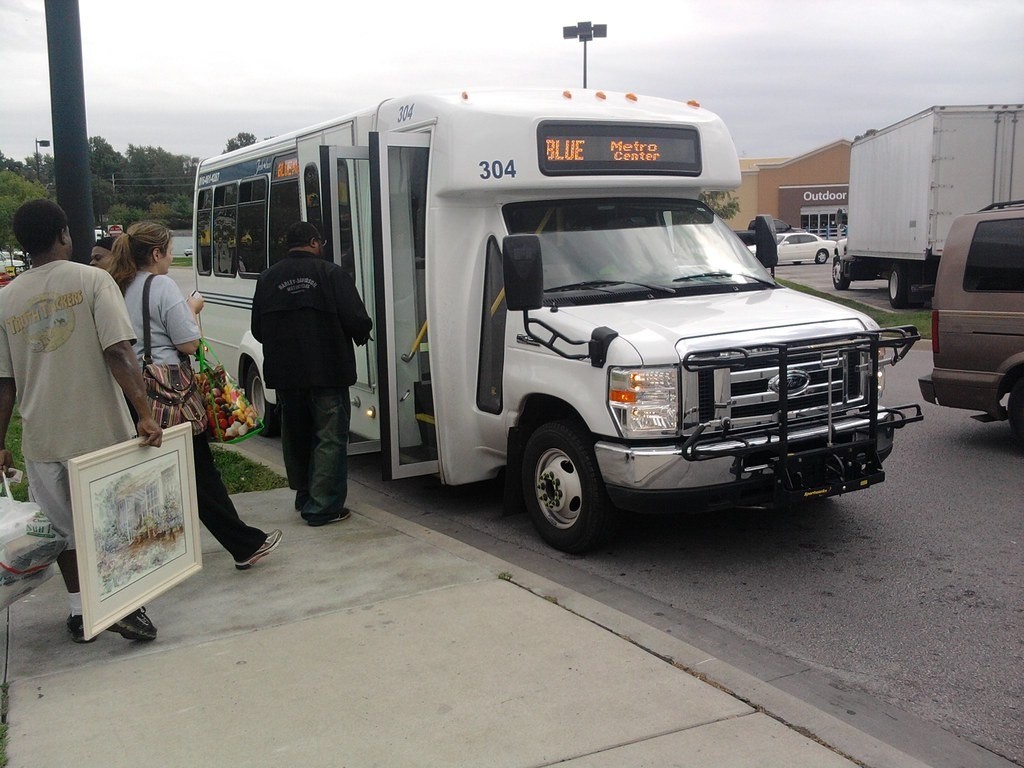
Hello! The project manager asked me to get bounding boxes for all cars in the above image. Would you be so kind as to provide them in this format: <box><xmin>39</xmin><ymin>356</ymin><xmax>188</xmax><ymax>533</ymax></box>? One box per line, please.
<box><xmin>747</xmin><ymin>219</ymin><xmax>806</xmax><ymax>233</ymax></box>
<box><xmin>96</xmin><ymin>229</ymin><xmax>106</xmax><ymax>241</ymax></box>
<box><xmin>747</xmin><ymin>232</ymin><xmax>835</xmax><ymax>265</ymax></box>
<box><xmin>0</xmin><ymin>251</ymin><xmax>33</xmax><ymax>278</ymax></box>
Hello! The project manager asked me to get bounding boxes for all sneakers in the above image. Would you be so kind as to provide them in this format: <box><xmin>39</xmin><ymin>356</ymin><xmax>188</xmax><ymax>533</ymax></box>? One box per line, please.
<box><xmin>235</xmin><ymin>529</ymin><xmax>283</xmax><ymax>570</ymax></box>
<box><xmin>67</xmin><ymin>612</ymin><xmax>97</xmax><ymax>643</ymax></box>
<box><xmin>105</xmin><ymin>606</ymin><xmax>157</xmax><ymax>640</ymax></box>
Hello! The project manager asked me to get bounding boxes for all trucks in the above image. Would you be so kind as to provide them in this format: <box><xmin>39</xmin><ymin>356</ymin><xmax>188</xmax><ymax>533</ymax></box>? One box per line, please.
<box><xmin>193</xmin><ymin>91</ymin><xmax>925</xmax><ymax>555</ymax></box>
<box><xmin>831</xmin><ymin>103</ymin><xmax>1024</xmax><ymax>309</ymax></box>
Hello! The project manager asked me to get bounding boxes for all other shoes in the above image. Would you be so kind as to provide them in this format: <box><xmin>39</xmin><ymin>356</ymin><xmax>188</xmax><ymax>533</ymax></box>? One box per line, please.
<box><xmin>308</xmin><ymin>508</ymin><xmax>350</xmax><ymax>527</ymax></box>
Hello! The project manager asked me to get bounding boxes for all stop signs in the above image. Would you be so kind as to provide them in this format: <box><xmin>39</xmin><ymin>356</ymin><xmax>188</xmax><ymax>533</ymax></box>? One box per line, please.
<box><xmin>107</xmin><ymin>225</ymin><xmax>124</xmax><ymax>239</ymax></box>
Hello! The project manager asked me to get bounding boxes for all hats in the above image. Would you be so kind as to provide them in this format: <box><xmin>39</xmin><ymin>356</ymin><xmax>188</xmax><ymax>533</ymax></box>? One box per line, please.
<box><xmin>94</xmin><ymin>237</ymin><xmax>116</xmax><ymax>251</ymax></box>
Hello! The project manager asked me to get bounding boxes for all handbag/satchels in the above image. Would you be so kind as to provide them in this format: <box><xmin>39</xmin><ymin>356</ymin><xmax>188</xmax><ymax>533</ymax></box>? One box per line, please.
<box><xmin>0</xmin><ymin>467</ymin><xmax>68</xmax><ymax>614</ymax></box>
<box><xmin>134</xmin><ymin>273</ymin><xmax>207</xmax><ymax>436</ymax></box>
<box><xmin>192</xmin><ymin>338</ymin><xmax>265</xmax><ymax>444</ymax></box>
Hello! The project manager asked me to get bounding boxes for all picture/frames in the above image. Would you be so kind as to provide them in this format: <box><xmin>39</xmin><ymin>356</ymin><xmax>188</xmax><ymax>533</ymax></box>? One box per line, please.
<box><xmin>67</xmin><ymin>422</ymin><xmax>204</xmax><ymax>641</ymax></box>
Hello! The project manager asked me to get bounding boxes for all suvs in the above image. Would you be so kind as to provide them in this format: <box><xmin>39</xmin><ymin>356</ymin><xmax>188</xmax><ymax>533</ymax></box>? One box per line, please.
<box><xmin>917</xmin><ymin>200</ymin><xmax>1024</xmax><ymax>444</ymax></box>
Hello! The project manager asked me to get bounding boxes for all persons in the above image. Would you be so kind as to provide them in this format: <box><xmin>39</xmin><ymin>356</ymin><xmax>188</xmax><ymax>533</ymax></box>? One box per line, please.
<box><xmin>598</xmin><ymin>231</ymin><xmax>672</xmax><ymax>278</ymax></box>
<box><xmin>251</xmin><ymin>222</ymin><xmax>374</xmax><ymax>527</ymax></box>
<box><xmin>0</xmin><ymin>199</ymin><xmax>282</xmax><ymax>644</ymax></box>
<box><xmin>238</xmin><ymin>239</ymin><xmax>286</xmax><ymax>272</ymax></box>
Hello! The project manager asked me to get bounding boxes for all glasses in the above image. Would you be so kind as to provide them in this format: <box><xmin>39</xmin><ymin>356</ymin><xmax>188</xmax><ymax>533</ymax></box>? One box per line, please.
<box><xmin>316</xmin><ymin>237</ymin><xmax>328</xmax><ymax>246</ymax></box>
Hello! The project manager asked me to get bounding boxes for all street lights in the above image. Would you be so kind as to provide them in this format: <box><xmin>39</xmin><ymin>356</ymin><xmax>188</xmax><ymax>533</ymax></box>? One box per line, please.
<box><xmin>564</xmin><ymin>22</ymin><xmax>610</xmax><ymax>92</ymax></box>
<box><xmin>35</xmin><ymin>138</ymin><xmax>50</xmax><ymax>180</ymax></box>
